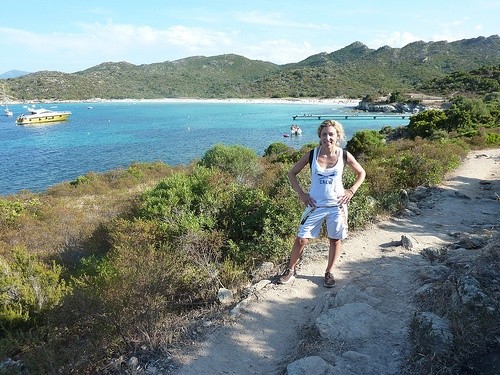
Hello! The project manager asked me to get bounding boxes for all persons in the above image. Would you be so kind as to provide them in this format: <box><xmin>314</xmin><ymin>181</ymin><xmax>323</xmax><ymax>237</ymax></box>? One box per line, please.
<box><xmin>291</xmin><ymin>124</ymin><xmax>299</xmax><ymax>132</ymax></box>
<box><xmin>277</xmin><ymin>119</ymin><xmax>366</xmax><ymax>287</ymax></box>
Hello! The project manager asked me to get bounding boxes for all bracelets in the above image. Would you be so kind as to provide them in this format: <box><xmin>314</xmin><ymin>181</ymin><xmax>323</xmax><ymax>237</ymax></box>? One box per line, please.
<box><xmin>349</xmin><ymin>189</ymin><xmax>354</xmax><ymax>195</ymax></box>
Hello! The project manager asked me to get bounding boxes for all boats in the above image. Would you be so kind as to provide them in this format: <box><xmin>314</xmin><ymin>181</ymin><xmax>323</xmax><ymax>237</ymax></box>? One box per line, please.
<box><xmin>291</xmin><ymin>125</ymin><xmax>302</xmax><ymax>135</ymax></box>
<box><xmin>15</xmin><ymin>108</ymin><xmax>73</xmax><ymax>125</ymax></box>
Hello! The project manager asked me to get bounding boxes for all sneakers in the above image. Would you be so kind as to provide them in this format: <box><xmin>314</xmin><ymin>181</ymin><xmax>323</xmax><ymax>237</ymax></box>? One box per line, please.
<box><xmin>276</xmin><ymin>268</ymin><xmax>298</xmax><ymax>284</ymax></box>
<box><xmin>323</xmin><ymin>272</ymin><xmax>336</xmax><ymax>288</ymax></box>
